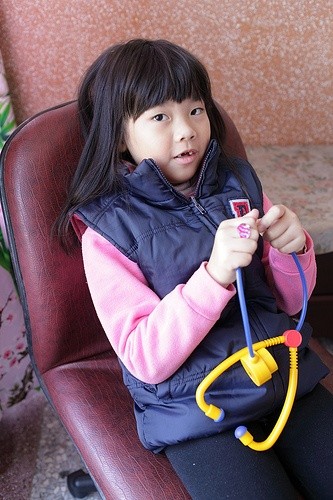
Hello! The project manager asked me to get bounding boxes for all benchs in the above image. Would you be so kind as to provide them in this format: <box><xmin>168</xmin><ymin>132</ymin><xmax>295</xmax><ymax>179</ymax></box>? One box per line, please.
<box><xmin>1</xmin><ymin>1</ymin><xmax>333</xmax><ymax>342</ymax></box>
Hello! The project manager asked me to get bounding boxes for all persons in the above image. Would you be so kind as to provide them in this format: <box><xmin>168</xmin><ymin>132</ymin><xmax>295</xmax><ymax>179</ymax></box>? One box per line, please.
<box><xmin>49</xmin><ymin>38</ymin><xmax>333</xmax><ymax>499</ymax></box>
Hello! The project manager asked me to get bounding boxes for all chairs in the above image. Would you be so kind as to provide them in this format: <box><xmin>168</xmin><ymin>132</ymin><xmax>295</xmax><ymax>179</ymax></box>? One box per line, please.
<box><xmin>0</xmin><ymin>98</ymin><xmax>248</xmax><ymax>500</ymax></box>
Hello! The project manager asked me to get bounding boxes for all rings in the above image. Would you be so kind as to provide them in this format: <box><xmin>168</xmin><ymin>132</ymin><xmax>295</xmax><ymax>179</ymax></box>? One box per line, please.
<box><xmin>237</xmin><ymin>222</ymin><xmax>250</xmax><ymax>239</ymax></box>
